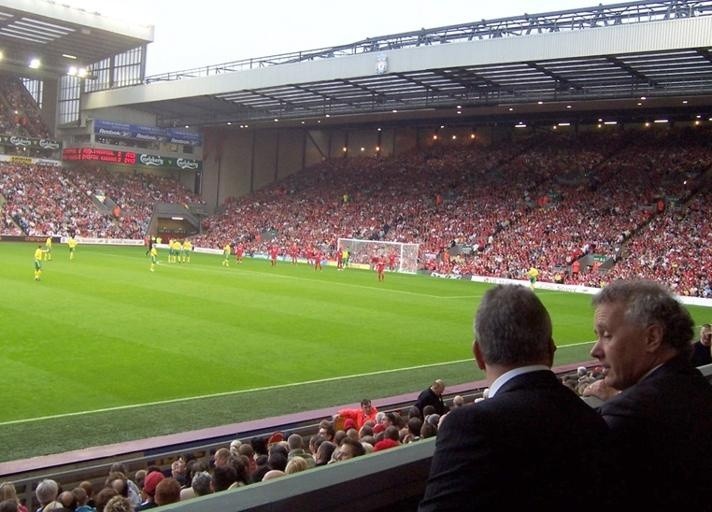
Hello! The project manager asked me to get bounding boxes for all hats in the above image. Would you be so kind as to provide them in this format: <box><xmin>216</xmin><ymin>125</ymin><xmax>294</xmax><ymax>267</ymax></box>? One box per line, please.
<box><xmin>344</xmin><ymin>419</ymin><xmax>356</xmax><ymax>430</ymax></box>
<box><xmin>144</xmin><ymin>471</ymin><xmax>164</xmax><ymax>495</ymax></box>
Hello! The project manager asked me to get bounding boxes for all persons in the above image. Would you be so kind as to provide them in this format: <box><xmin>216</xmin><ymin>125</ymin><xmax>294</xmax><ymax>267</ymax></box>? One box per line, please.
<box><xmin>1</xmin><ymin>378</ymin><xmax>445</xmax><ymax>511</ymax></box>
<box><xmin>420</xmin><ymin>278</ymin><xmax>712</xmax><ymax>512</ymax></box>
<box><xmin>1</xmin><ymin>69</ymin><xmax>712</xmax><ymax>297</ymax></box>
